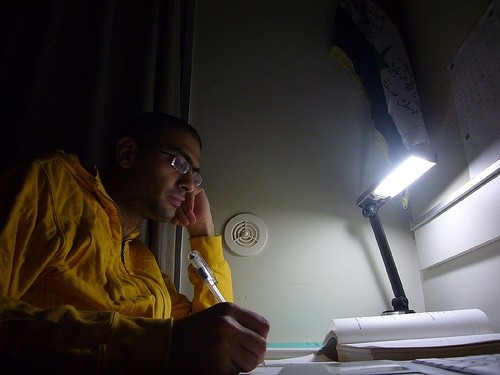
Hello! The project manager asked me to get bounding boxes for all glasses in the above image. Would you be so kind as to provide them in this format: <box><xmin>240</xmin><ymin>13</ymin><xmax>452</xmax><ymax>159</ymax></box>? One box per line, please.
<box><xmin>143</xmin><ymin>148</ymin><xmax>203</xmax><ymax>187</ymax></box>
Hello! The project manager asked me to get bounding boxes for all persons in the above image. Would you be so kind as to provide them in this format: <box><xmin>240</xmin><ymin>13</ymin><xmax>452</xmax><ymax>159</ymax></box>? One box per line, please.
<box><xmin>0</xmin><ymin>112</ymin><xmax>270</xmax><ymax>375</ymax></box>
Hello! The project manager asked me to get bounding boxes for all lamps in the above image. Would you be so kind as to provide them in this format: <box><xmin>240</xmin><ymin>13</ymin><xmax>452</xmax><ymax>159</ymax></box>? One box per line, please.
<box><xmin>356</xmin><ymin>145</ymin><xmax>438</xmax><ymax>316</ymax></box>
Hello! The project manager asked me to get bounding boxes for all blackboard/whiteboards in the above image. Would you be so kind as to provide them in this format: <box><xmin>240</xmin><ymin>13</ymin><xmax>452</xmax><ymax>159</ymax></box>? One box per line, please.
<box><xmin>393</xmin><ymin>5</ymin><xmax>500</xmax><ymax>236</ymax></box>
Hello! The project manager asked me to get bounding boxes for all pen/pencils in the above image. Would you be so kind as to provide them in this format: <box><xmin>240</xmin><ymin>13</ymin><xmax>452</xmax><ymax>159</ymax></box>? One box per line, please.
<box><xmin>187</xmin><ymin>250</ymin><xmax>268</xmax><ymax>369</ymax></box>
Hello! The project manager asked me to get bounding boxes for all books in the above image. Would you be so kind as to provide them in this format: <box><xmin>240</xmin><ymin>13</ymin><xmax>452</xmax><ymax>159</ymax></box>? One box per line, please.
<box><xmin>238</xmin><ymin>308</ymin><xmax>500</xmax><ymax>375</ymax></box>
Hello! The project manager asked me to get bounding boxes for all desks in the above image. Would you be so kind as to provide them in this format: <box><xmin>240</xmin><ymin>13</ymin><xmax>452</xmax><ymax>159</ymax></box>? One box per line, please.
<box><xmin>239</xmin><ymin>350</ymin><xmax>499</xmax><ymax>374</ymax></box>
